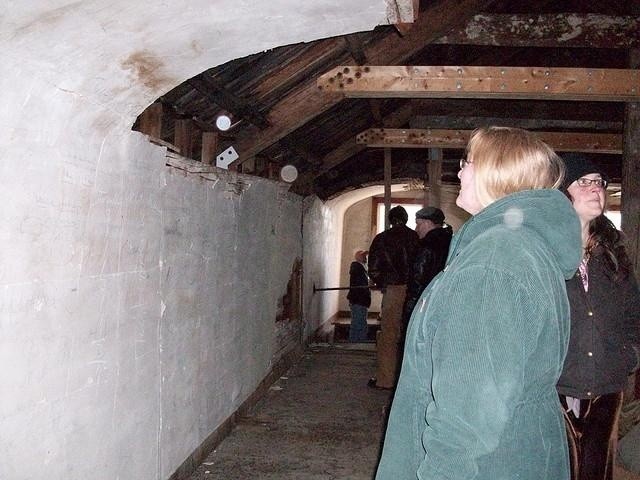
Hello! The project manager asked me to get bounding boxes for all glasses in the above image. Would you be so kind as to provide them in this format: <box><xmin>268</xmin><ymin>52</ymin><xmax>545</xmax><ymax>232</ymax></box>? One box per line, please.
<box><xmin>458</xmin><ymin>158</ymin><xmax>475</xmax><ymax>168</ymax></box>
<box><xmin>578</xmin><ymin>178</ymin><xmax>608</xmax><ymax>189</ymax></box>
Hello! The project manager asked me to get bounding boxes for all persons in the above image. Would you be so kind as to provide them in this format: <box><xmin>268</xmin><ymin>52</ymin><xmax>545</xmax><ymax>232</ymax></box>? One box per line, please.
<box><xmin>368</xmin><ymin>208</ymin><xmax>420</xmax><ymax>390</ymax></box>
<box><xmin>346</xmin><ymin>249</ymin><xmax>370</xmax><ymax>341</ymax></box>
<box><xmin>373</xmin><ymin>125</ymin><xmax>582</xmax><ymax>480</ymax></box>
<box><xmin>400</xmin><ymin>206</ymin><xmax>452</xmax><ymax>333</ymax></box>
<box><xmin>552</xmin><ymin>153</ymin><xmax>640</xmax><ymax>480</ymax></box>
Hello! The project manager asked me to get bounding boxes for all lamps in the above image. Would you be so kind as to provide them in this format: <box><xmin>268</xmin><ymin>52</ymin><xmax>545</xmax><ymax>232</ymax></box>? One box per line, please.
<box><xmin>216</xmin><ymin>111</ymin><xmax>234</xmax><ymax>131</ymax></box>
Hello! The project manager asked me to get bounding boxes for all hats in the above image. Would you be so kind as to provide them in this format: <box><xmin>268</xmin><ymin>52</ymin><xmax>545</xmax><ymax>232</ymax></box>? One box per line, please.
<box><xmin>562</xmin><ymin>154</ymin><xmax>603</xmax><ymax>191</ymax></box>
<box><xmin>416</xmin><ymin>206</ymin><xmax>445</xmax><ymax>223</ymax></box>
<box><xmin>355</xmin><ymin>250</ymin><xmax>368</xmax><ymax>257</ymax></box>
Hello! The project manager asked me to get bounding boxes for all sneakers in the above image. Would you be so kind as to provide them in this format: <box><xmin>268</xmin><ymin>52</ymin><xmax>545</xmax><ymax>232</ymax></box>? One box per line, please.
<box><xmin>367</xmin><ymin>377</ymin><xmax>386</xmax><ymax>389</ymax></box>
